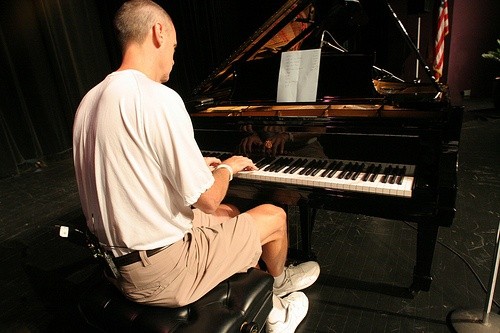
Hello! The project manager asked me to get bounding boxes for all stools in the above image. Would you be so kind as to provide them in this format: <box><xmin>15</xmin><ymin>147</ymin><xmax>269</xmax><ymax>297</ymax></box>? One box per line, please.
<box><xmin>77</xmin><ymin>268</ymin><xmax>274</xmax><ymax>333</ymax></box>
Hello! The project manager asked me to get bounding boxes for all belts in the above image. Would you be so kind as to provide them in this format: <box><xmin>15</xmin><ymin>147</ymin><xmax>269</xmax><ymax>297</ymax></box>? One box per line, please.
<box><xmin>103</xmin><ymin>243</ymin><xmax>173</xmax><ymax>269</ymax></box>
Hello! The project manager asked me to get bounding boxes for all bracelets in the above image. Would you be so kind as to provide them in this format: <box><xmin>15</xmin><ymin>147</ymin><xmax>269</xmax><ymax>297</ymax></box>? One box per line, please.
<box><xmin>213</xmin><ymin>164</ymin><xmax>233</xmax><ymax>182</ymax></box>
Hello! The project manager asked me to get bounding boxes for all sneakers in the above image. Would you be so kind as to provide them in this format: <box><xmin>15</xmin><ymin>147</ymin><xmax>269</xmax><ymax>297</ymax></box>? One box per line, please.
<box><xmin>266</xmin><ymin>292</ymin><xmax>310</xmax><ymax>333</ymax></box>
<box><xmin>273</xmin><ymin>261</ymin><xmax>320</xmax><ymax>298</ymax></box>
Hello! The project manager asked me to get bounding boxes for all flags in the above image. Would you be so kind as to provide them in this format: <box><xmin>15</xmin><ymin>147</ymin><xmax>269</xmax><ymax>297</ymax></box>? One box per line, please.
<box><xmin>424</xmin><ymin>0</ymin><xmax>449</xmax><ymax>82</ymax></box>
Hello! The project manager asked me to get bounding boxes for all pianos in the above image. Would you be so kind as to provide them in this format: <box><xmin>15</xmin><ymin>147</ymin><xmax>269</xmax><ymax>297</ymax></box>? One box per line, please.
<box><xmin>176</xmin><ymin>1</ymin><xmax>465</xmax><ymax>295</ymax></box>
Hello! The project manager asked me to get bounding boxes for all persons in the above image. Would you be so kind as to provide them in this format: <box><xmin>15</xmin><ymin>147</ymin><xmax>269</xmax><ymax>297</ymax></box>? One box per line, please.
<box><xmin>73</xmin><ymin>0</ymin><xmax>321</xmax><ymax>333</ymax></box>
